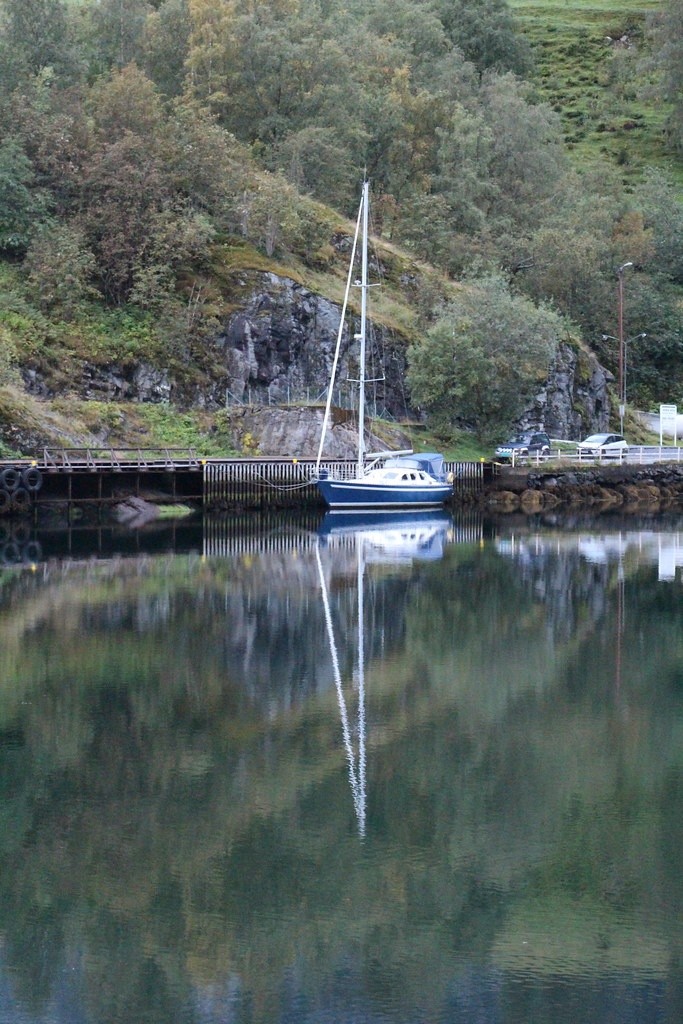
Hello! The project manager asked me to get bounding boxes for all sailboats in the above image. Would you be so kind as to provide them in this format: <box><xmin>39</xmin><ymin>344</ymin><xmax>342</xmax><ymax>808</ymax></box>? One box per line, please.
<box><xmin>303</xmin><ymin>522</ymin><xmax>447</xmax><ymax>844</ymax></box>
<box><xmin>309</xmin><ymin>177</ymin><xmax>458</xmax><ymax>509</ymax></box>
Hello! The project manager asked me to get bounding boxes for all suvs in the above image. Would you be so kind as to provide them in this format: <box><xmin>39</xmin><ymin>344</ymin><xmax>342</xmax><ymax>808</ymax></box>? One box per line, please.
<box><xmin>495</xmin><ymin>431</ymin><xmax>551</xmax><ymax>463</ymax></box>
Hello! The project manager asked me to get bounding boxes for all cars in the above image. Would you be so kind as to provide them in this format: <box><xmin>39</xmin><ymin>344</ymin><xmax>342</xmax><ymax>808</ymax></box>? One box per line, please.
<box><xmin>576</xmin><ymin>432</ymin><xmax>629</xmax><ymax>458</ymax></box>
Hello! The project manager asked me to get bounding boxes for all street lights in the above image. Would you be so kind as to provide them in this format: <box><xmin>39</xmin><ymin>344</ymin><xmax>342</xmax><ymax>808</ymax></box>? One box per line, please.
<box><xmin>603</xmin><ymin>332</ymin><xmax>646</xmax><ymax>435</ymax></box>
<box><xmin>618</xmin><ymin>259</ymin><xmax>634</xmax><ymax>431</ymax></box>
